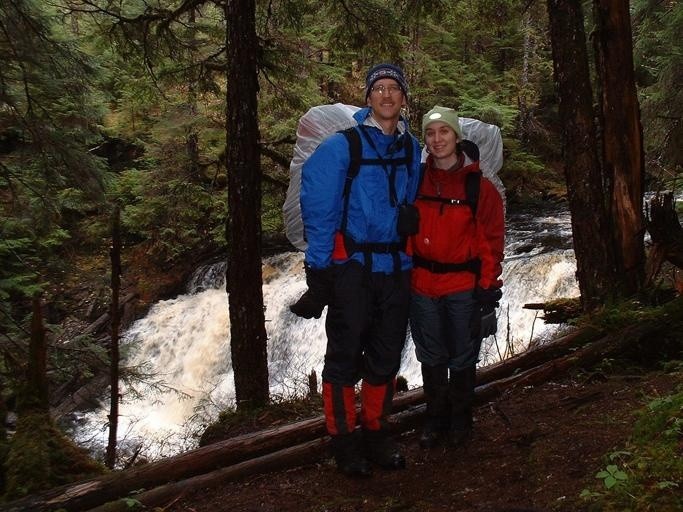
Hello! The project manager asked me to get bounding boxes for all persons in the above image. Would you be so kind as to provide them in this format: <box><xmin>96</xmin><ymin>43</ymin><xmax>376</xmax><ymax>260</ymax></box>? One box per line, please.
<box><xmin>289</xmin><ymin>64</ymin><xmax>426</xmax><ymax>480</ymax></box>
<box><xmin>402</xmin><ymin>104</ymin><xmax>504</xmax><ymax>446</ymax></box>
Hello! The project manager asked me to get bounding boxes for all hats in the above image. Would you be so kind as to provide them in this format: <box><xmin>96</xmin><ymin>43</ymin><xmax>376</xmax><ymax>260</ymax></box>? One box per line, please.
<box><xmin>366</xmin><ymin>64</ymin><xmax>407</xmax><ymax>104</ymax></box>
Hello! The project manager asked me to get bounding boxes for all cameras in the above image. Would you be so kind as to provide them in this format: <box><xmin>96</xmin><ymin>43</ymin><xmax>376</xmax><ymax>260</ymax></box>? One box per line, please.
<box><xmin>397</xmin><ymin>204</ymin><xmax>419</xmax><ymax>236</ymax></box>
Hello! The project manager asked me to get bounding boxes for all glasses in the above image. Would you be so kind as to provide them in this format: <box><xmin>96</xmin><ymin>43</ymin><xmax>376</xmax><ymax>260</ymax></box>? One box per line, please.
<box><xmin>371</xmin><ymin>84</ymin><xmax>402</xmax><ymax>95</ymax></box>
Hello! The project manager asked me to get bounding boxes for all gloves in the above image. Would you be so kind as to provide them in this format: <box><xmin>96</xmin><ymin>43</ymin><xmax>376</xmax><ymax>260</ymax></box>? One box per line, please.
<box><xmin>468</xmin><ymin>306</ymin><xmax>497</xmax><ymax>339</ymax></box>
<box><xmin>289</xmin><ymin>287</ymin><xmax>325</xmax><ymax>319</ymax></box>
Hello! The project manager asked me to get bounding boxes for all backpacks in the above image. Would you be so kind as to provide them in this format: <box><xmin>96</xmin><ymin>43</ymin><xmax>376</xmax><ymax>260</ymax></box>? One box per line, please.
<box><xmin>282</xmin><ymin>103</ymin><xmax>506</xmax><ymax>251</ymax></box>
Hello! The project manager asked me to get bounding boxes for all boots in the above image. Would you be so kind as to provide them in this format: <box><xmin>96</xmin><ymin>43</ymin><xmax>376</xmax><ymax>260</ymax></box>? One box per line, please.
<box><xmin>322</xmin><ymin>379</ymin><xmax>405</xmax><ymax>478</ymax></box>
<box><xmin>419</xmin><ymin>362</ymin><xmax>476</xmax><ymax>447</ymax></box>
